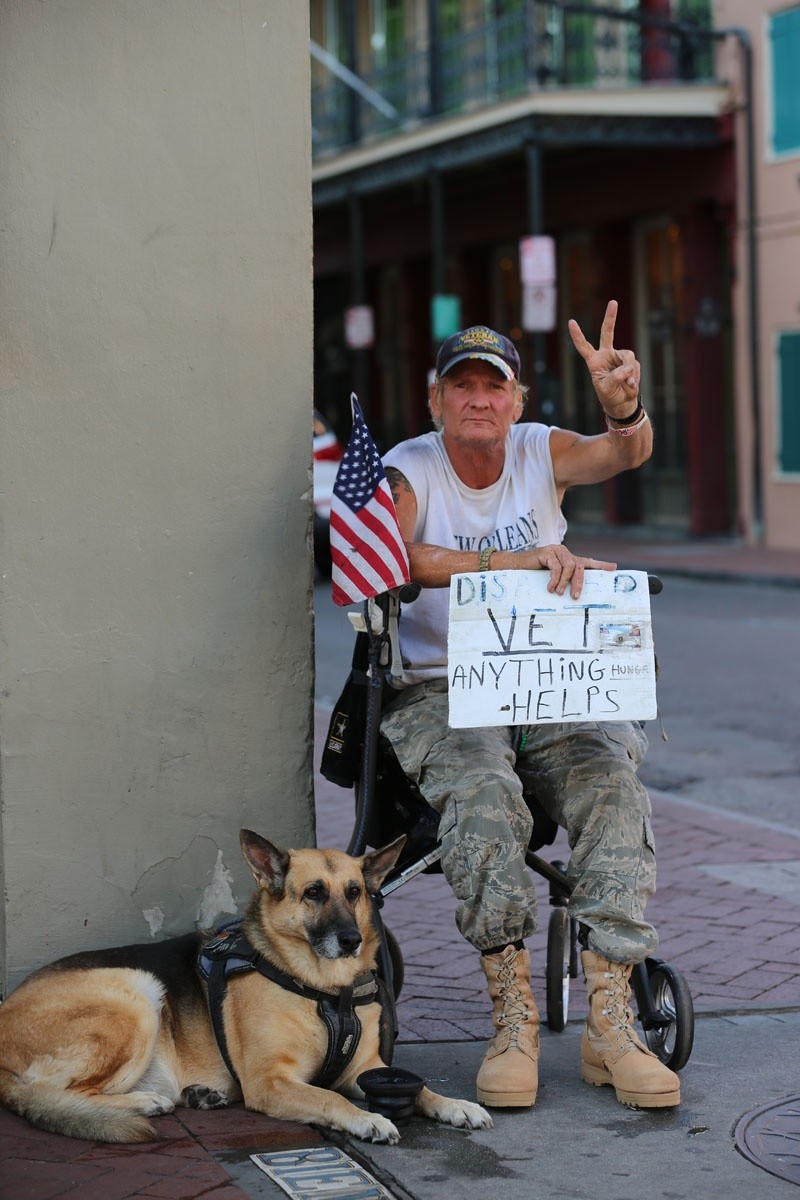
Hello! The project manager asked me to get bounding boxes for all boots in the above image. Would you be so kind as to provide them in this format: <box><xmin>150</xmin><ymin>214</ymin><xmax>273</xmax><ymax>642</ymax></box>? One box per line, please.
<box><xmin>580</xmin><ymin>951</ymin><xmax>682</xmax><ymax>1108</ymax></box>
<box><xmin>477</xmin><ymin>946</ymin><xmax>541</xmax><ymax>1108</ymax></box>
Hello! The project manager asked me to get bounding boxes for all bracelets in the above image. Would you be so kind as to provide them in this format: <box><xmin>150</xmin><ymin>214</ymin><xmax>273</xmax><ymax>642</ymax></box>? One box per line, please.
<box><xmin>480</xmin><ymin>546</ymin><xmax>498</xmax><ymax>571</ymax></box>
<box><xmin>605</xmin><ymin>410</ymin><xmax>648</xmax><ymax>436</ymax></box>
<box><xmin>605</xmin><ymin>391</ymin><xmax>643</xmax><ymax>424</ymax></box>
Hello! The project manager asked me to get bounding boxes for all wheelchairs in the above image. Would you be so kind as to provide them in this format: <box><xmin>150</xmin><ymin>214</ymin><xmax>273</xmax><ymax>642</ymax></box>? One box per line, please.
<box><xmin>345</xmin><ymin>580</ymin><xmax>697</xmax><ymax>1074</ymax></box>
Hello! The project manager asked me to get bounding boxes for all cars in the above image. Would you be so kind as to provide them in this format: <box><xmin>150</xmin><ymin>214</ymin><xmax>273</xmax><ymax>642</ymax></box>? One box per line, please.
<box><xmin>312</xmin><ymin>407</ymin><xmax>343</xmax><ymax>578</ymax></box>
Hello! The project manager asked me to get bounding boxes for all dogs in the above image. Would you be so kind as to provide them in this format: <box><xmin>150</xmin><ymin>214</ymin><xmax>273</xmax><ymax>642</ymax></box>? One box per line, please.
<box><xmin>2</xmin><ymin>830</ymin><xmax>495</xmax><ymax>1144</ymax></box>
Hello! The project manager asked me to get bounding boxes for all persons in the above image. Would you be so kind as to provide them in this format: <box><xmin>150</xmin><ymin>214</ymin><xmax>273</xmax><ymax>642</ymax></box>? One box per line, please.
<box><xmin>376</xmin><ymin>303</ymin><xmax>682</xmax><ymax>1109</ymax></box>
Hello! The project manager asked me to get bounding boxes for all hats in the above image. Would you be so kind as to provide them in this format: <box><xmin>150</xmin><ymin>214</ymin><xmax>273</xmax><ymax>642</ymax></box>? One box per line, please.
<box><xmin>436</xmin><ymin>325</ymin><xmax>521</xmax><ymax>382</ymax></box>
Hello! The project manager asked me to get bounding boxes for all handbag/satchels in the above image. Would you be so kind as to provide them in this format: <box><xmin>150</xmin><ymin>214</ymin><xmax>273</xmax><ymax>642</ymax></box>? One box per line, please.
<box><xmin>319</xmin><ymin>631</ymin><xmax>368</xmax><ymax>788</ymax></box>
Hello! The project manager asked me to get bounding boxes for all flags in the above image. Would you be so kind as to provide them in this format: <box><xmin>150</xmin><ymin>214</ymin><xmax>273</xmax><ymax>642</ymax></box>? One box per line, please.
<box><xmin>330</xmin><ymin>392</ymin><xmax>412</xmax><ymax>607</ymax></box>
